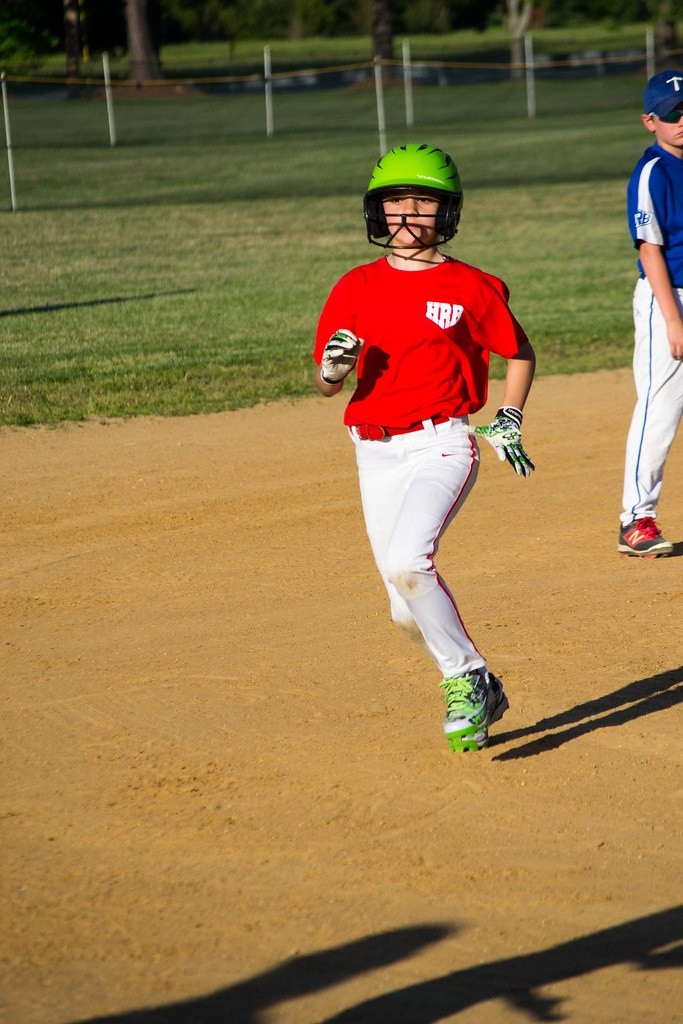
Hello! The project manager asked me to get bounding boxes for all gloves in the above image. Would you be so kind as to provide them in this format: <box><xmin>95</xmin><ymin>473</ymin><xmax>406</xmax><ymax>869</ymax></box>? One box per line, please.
<box><xmin>464</xmin><ymin>406</ymin><xmax>536</xmax><ymax>478</ymax></box>
<box><xmin>320</xmin><ymin>329</ymin><xmax>365</xmax><ymax>385</ymax></box>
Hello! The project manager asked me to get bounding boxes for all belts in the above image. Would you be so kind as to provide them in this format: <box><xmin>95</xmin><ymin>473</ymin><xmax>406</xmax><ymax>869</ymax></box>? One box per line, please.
<box><xmin>350</xmin><ymin>415</ymin><xmax>450</xmax><ymax>441</ymax></box>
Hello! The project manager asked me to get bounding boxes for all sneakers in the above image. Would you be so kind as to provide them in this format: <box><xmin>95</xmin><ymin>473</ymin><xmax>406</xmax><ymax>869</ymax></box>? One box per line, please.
<box><xmin>448</xmin><ymin>691</ymin><xmax>509</xmax><ymax>751</ymax></box>
<box><xmin>618</xmin><ymin>516</ymin><xmax>673</xmax><ymax>559</ymax></box>
<box><xmin>439</xmin><ymin>668</ymin><xmax>503</xmax><ymax>740</ymax></box>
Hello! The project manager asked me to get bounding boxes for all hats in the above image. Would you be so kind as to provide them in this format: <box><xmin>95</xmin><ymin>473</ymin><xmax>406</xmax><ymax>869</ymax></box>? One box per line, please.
<box><xmin>642</xmin><ymin>70</ymin><xmax>683</xmax><ymax>118</ymax></box>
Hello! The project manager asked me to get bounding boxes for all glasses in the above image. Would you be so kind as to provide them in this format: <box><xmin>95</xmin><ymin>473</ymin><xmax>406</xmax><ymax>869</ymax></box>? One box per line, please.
<box><xmin>646</xmin><ymin>108</ymin><xmax>683</xmax><ymax>123</ymax></box>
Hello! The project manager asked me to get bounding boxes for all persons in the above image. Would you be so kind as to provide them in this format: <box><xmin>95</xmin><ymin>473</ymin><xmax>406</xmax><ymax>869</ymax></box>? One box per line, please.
<box><xmin>617</xmin><ymin>68</ymin><xmax>683</xmax><ymax>560</ymax></box>
<box><xmin>313</xmin><ymin>144</ymin><xmax>539</xmax><ymax>755</ymax></box>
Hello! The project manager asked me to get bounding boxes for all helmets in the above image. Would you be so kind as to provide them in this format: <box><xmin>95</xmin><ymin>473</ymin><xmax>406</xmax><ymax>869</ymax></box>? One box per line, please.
<box><xmin>364</xmin><ymin>143</ymin><xmax>463</xmax><ymax>239</ymax></box>
